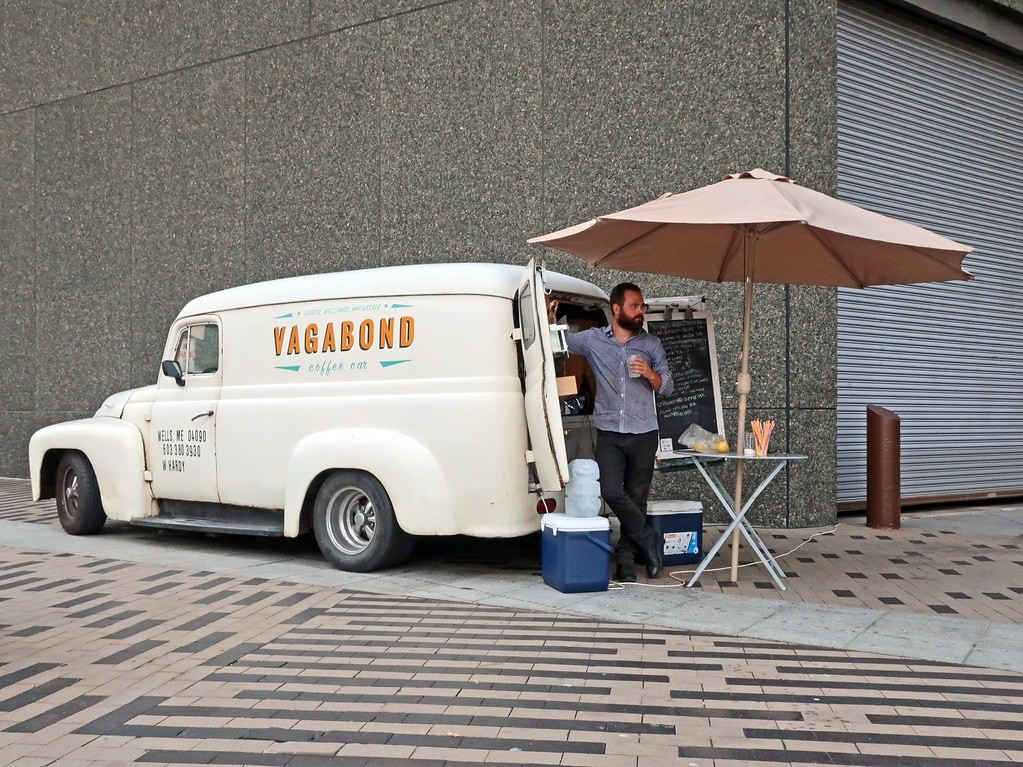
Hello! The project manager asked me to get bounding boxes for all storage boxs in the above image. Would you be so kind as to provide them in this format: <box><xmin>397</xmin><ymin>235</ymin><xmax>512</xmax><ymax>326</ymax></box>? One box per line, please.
<box><xmin>540</xmin><ymin>513</ymin><xmax>612</xmax><ymax>593</ymax></box>
<box><xmin>635</xmin><ymin>500</ymin><xmax>704</xmax><ymax>566</ymax></box>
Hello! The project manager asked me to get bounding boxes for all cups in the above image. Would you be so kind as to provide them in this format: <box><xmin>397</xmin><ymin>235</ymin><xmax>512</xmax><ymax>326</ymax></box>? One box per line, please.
<box><xmin>744</xmin><ymin>432</ymin><xmax>755</xmax><ymax>456</ymax></box>
<box><xmin>756</xmin><ymin>435</ymin><xmax>770</xmax><ymax>457</ymax></box>
<box><xmin>625</xmin><ymin>354</ymin><xmax>642</xmax><ymax>379</ymax></box>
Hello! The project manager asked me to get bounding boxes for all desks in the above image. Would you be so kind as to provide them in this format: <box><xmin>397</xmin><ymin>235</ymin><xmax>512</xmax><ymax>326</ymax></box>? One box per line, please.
<box><xmin>674</xmin><ymin>449</ymin><xmax>809</xmax><ymax>591</ymax></box>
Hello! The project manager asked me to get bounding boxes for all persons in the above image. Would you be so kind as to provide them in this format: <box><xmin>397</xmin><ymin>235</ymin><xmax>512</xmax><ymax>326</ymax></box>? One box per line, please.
<box><xmin>546</xmin><ymin>282</ymin><xmax>675</xmax><ymax>581</ymax></box>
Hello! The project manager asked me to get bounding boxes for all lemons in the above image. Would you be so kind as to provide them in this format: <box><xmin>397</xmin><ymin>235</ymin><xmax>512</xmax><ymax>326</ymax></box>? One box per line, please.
<box><xmin>717</xmin><ymin>442</ymin><xmax>730</xmax><ymax>453</ymax></box>
<box><xmin>694</xmin><ymin>443</ymin><xmax>705</xmax><ymax>452</ymax></box>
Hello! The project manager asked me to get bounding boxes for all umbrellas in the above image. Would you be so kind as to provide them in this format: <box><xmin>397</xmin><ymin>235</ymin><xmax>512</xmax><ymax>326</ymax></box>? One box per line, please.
<box><xmin>527</xmin><ymin>168</ymin><xmax>975</xmax><ymax>585</ymax></box>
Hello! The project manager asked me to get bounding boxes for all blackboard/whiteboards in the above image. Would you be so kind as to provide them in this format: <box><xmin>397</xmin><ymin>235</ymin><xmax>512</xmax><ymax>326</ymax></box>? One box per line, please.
<box><xmin>641</xmin><ymin>310</ymin><xmax>723</xmax><ymax>460</ymax></box>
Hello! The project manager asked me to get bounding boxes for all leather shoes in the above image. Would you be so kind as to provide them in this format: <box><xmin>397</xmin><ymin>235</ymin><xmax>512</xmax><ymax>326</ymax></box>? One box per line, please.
<box><xmin>646</xmin><ymin>532</ymin><xmax>665</xmax><ymax>577</ymax></box>
<box><xmin>616</xmin><ymin>557</ymin><xmax>637</xmax><ymax>582</ymax></box>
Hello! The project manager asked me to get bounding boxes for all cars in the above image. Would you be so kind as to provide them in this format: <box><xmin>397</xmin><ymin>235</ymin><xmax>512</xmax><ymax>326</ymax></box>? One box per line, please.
<box><xmin>24</xmin><ymin>258</ymin><xmax>614</xmax><ymax>575</ymax></box>
